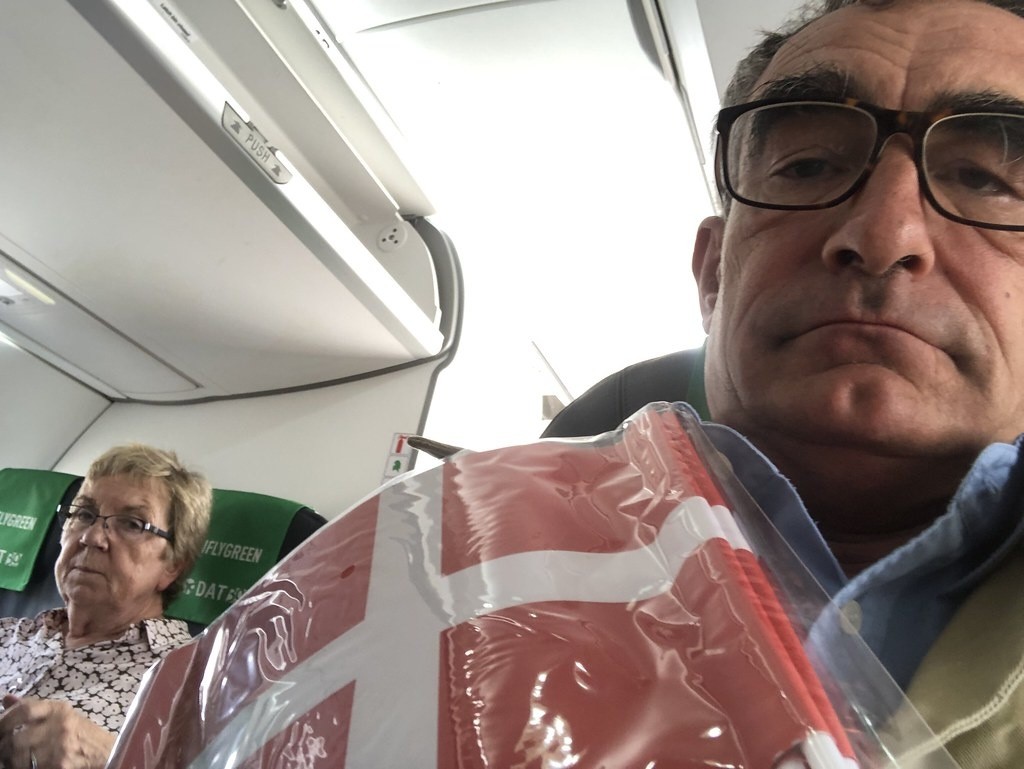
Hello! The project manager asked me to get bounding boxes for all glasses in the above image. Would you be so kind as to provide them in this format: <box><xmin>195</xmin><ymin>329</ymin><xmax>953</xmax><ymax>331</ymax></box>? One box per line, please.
<box><xmin>709</xmin><ymin>95</ymin><xmax>1024</xmax><ymax>231</ymax></box>
<box><xmin>56</xmin><ymin>503</ymin><xmax>172</xmax><ymax>545</ymax></box>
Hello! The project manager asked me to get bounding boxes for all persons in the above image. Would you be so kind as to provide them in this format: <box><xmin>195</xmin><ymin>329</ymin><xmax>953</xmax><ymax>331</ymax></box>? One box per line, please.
<box><xmin>0</xmin><ymin>442</ymin><xmax>214</xmax><ymax>769</ymax></box>
<box><xmin>114</xmin><ymin>0</ymin><xmax>1024</xmax><ymax>769</ymax></box>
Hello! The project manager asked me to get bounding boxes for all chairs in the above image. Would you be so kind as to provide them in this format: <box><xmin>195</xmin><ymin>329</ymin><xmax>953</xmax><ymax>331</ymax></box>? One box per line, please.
<box><xmin>0</xmin><ymin>466</ymin><xmax>89</xmax><ymax>621</ymax></box>
<box><xmin>160</xmin><ymin>487</ymin><xmax>330</xmax><ymax>637</ymax></box>
